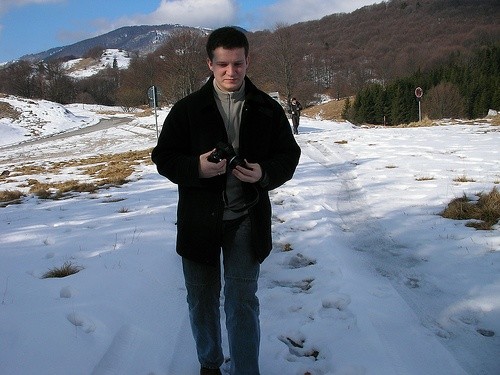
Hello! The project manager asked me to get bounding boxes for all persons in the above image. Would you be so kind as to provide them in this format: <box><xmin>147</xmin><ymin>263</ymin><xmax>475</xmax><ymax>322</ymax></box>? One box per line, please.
<box><xmin>149</xmin><ymin>27</ymin><xmax>304</xmax><ymax>375</ymax></box>
<box><xmin>287</xmin><ymin>97</ymin><xmax>302</xmax><ymax>135</ymax></box>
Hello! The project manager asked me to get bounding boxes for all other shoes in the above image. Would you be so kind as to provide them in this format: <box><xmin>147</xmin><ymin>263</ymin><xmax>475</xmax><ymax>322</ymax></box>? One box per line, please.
<box><xmin>200</xmin><ymin>366</ymin><xmax>221</xmax><ymax>375</ymax></box>
<box><xmin>293</xmin><ymin>128</ymin><xmax>298</xmax><ymax>135</ymax></box>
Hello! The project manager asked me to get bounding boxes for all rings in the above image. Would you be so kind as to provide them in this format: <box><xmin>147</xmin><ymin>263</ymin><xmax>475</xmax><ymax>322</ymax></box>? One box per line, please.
<box><xmin>218</xmin><ymin>173</ymin><xmax>221</xmax><ymax>176</ymax></box>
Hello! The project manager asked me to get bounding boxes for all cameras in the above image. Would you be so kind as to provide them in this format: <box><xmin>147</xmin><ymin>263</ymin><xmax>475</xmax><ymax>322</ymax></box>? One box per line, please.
<box><xmin>208</xmin><ymin>143</ymin><xmax>246</xmax><ymax>171</ymax></box>
